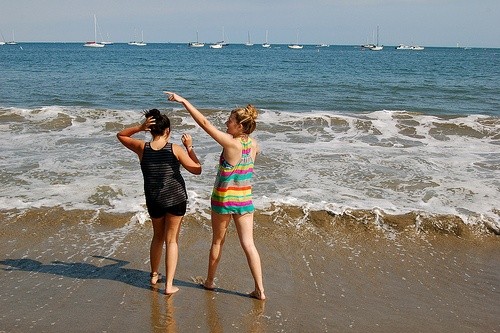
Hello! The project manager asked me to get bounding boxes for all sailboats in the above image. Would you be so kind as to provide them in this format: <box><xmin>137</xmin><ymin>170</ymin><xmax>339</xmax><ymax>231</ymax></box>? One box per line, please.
<box><xmin>7</xmin><ymin>31</ymin><xmax>18</xmax><ymax>45</ymax></box>
<box><xmin>191</xmin><ymin>32</ymin><xmax>205</xmax><ymax>48</ymax></box>
<box><xmin>99</xmin><ymin>32</ymin><xmax>113</xmax><ymax>45</ymax></box>
<box><xmin>288</xmin><ymin>32</ymin><xmax>304</xmax><ymax>49</ymax></box>
<box><xmin>361</xmin><ymin>25</ymin><xmax>383</xmax><ymax>51</ymax></box>
<box><xmin>209</xmin><ymin>44</ymin><xmax>223</xmax><ymax>49</ymax></box>
<box><xmin>217</xmin><ymin>29</ymin><xmax>229</xmax><ymax>46</ymax></box>
<box><xmin>261</xmin><ymin>31</ymin><xmax>271</xmax><ymax>48</ymax></box>
<box><xmin>127</xmin><ymin>28</ymin><xmax>147</xmax><ymax>46</ymax></box>
<box><xmin>82</xmin><ymin>13</ymin><xmax>105</xmax><ymax>48</ymax></box>
<box><xmin>244</xmin><ymin>32</ymin><xmax>254</xmax><ymax>46</ymax></box>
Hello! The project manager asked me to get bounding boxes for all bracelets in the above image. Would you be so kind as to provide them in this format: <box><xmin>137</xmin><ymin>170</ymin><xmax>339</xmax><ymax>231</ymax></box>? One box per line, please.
<box><xmin>186</xmin><ymin>145</ymin><xmax>192</xmax><ymax>149</ymax></box>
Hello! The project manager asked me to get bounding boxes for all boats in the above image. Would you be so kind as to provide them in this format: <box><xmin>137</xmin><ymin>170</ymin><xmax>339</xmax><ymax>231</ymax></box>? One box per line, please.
<box><xmin>395</xmin><ymin>43</ymin><xmax>425</xmax><ymax>50</ymax></box>
<box><xmin>0</xmin><ymin>42</ymin><xmax>6</xmax><ymax>46</ymax></box>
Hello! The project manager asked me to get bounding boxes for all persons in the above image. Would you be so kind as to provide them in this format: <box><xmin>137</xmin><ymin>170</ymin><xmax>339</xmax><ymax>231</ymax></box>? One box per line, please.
<box><xmin>118</xmin><ymin>110</ymin><xmax>202</xmax><ymax>294</ymax></box>
<box><xmin>164</xmin><ymin>90</ymin><xmax>266</xmax><ymax>300</ymax></box>
